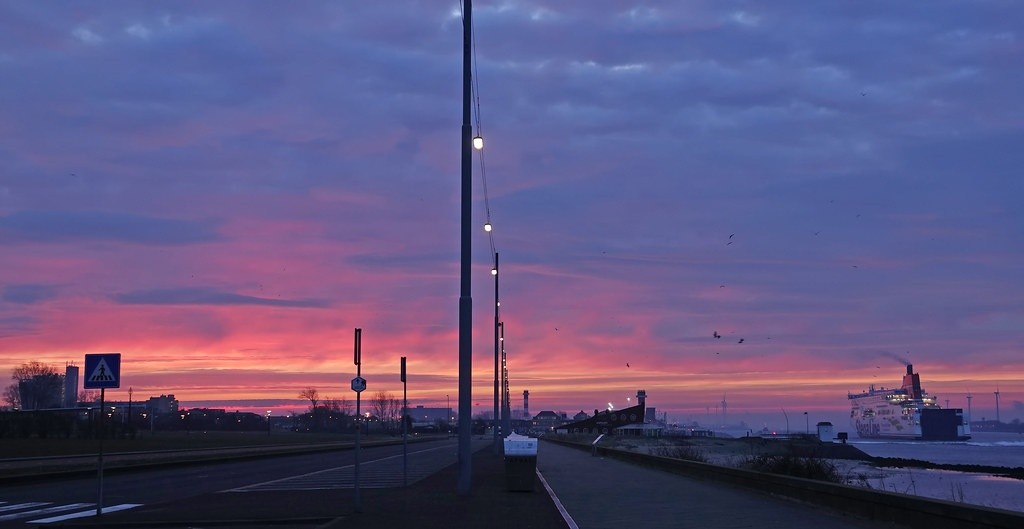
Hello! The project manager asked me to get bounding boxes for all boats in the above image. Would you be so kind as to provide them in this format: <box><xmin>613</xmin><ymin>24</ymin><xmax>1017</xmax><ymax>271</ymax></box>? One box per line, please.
<box><xmin>846</xmin><ymin>364</ymin><xmax>973</xmax><ymax>442</ymax></box>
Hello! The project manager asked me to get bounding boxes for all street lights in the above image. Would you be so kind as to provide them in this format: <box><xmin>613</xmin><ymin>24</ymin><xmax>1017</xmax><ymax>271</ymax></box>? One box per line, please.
<box><xmin>266</xmin><ymin>410</ymin><xmax>271</xmax><ymax>435</ymax></box>
<box><xmin>128</xmin><ymin>387</ymin><xmax>133</xmax><ymax>426</ymax></box>
<box><xmin>967</xmin><ymin>396</ymin><xmax>973</xmax><ymax>424</ymax></box>
<box><xmin>804</xmin><ymin>411</ymin><xmax>808</xmax><ymax>436</ymax></box>
<box><xmin>994</xmin><ymin>392</ymin><xmax>999</xmax><ymax>423</ymax></box>
<box><xmin>365</xmin><ymin>412</ymin><xmax>370</xmax><ymax>439</ymax></box>
<box><xmin>447</xmin><ymin>395</ymin><xmax>449</xmax><ymax>424</ymax></box>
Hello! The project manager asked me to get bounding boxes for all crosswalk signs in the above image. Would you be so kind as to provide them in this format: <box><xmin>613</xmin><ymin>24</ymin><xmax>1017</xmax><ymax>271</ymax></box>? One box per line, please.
<box><xmin>83</xmin><ymin>353</ymin><xmax>121</xmax><ymax>388</ymax></box>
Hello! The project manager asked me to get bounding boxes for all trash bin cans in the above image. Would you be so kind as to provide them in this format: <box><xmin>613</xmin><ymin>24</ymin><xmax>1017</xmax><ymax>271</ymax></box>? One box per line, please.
<box><xmin>503</xmin><ymin>437</ymin><xmax>538</xmax><ymax>492</ymax></box>
